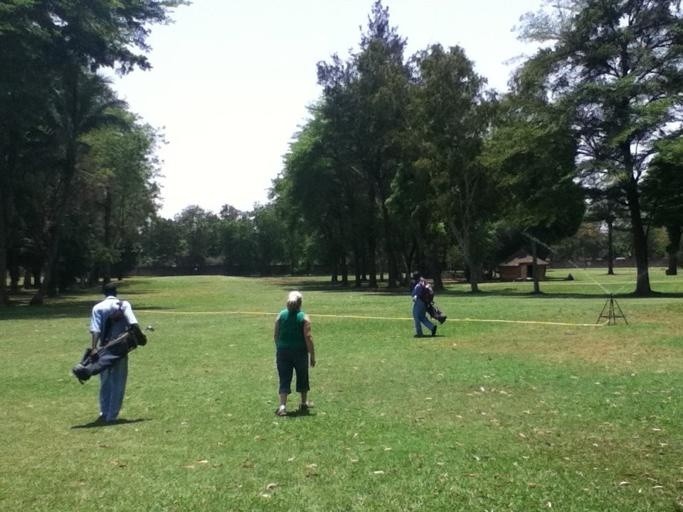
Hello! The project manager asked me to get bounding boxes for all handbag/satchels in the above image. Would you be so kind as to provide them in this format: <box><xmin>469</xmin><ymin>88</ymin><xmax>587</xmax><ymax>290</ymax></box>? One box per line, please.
<box><xmin>72</xmin><ymin>301</ymin><xmax>146</xmax><ymax>384</ymax></box>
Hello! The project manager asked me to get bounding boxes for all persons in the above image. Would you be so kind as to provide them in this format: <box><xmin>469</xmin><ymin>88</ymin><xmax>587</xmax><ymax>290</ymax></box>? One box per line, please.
<box><xmin>408</xmin><ymin>272</ymin><xmax>437</xmax><ymax>337</ymax></box>
<box><xmin>271</xmin><ymin>290</ymin><xmax>316</xmax><ymax>414</ymax></box>
<box><xmin>85</xmin><ymin>282</ymin><xmax>146</xmax><ymax>419</ymax></box>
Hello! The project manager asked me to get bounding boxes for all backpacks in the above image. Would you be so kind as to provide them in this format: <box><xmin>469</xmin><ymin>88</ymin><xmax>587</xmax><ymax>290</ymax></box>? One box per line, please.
<box><xmin>419</xmin><ymin>282</ymin><xmax>447</xmax><ymax>324</ymax></box>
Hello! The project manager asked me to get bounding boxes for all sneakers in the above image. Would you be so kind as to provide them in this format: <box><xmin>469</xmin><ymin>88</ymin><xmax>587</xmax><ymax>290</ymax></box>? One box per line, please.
<box><xmin>414</xmin><ymin>334</ymin><xmax>423</xmax><ymax>337</ymax></box>
<box><xmin>432</xmin><ymin>325</ymin><xmax>437</xmax><ymax>336</ymax></box>
<box><xmin>300</xmin><ymin>401</ymin><xmax>314</xmax><ymax>408</ymax></box>
<box><xmin>276</xmin><ymin>405</ymin><xmax>286</xmax><ymax>416</ymax></box>
